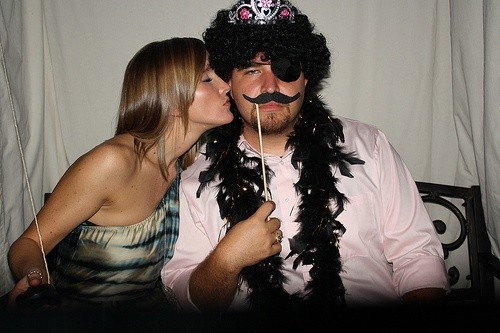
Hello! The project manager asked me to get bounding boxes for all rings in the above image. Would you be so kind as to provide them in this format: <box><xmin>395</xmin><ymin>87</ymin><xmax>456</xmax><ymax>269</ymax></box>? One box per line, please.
<box><xmin>27</xmin><ymin>270</ymin><xmax>43</xmax><ymax>280</ymax></box>
<box><xmin>273</xmin><ymin>232</ymin><xmax>283</xmax><ymax>245</ymax></box>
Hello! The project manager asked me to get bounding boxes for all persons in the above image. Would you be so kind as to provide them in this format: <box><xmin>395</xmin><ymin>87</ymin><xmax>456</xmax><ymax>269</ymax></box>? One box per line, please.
<box><xmin>161</xmin><ymin>0</ymin><xmax>453</xmax><ymax>306</ymax></box>
<box><xmin>9</xmin><ymin>38</ymin><xmax>235</xmax><ymax>306</ymax></box>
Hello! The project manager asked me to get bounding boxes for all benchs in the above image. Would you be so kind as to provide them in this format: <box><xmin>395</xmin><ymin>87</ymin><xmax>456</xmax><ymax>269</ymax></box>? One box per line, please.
<box><xmin>44</xmin><ymin>181</ymin><xmax>500</xmax><ymax>291</ymax></box>
